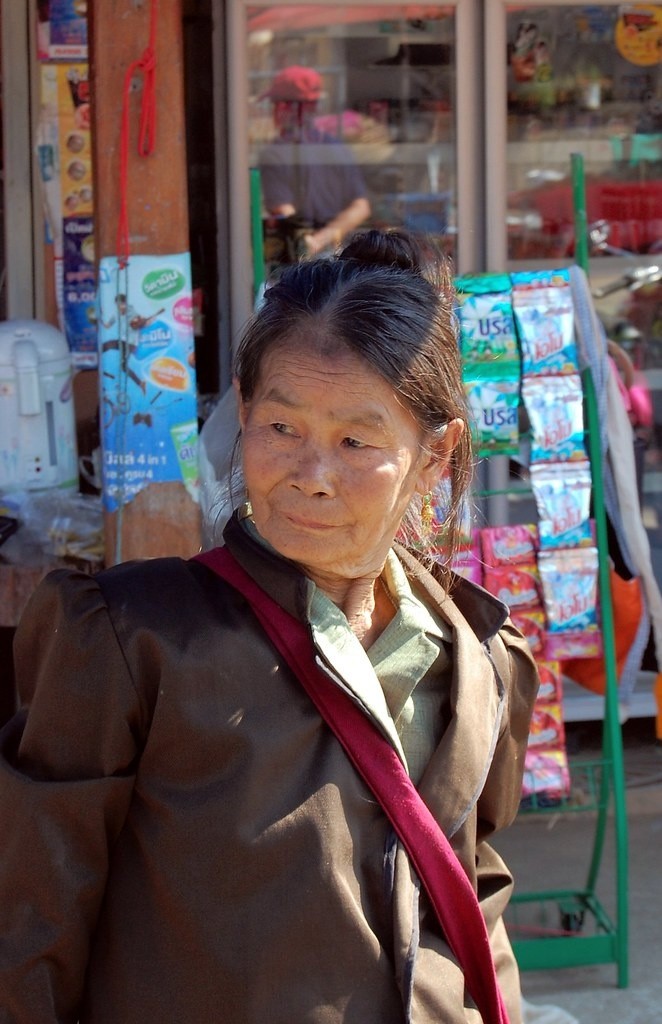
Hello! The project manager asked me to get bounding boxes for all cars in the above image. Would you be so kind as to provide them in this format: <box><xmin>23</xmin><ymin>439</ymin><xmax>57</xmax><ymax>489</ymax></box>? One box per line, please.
<box><xmin>501</xmin><ymin>160</ymin><xmax>662</xmax><ymax>258</ymax></box>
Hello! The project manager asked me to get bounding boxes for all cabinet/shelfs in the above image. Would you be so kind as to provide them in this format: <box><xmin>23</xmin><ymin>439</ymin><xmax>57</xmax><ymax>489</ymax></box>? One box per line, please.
<box><xmin>249</xmin><ymin>154</ymin><xmax>629</xmax><ymax>989</ymax></box>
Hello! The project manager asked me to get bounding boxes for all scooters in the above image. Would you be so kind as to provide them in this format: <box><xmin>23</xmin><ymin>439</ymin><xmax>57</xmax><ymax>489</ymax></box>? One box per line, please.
<box><xmin>587</xmin><ymin>217</ymin><xmax>662</xmax><ymax>370</ymax></box>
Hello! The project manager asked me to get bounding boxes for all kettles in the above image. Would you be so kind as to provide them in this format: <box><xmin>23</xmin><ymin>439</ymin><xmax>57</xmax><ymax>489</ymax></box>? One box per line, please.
<box><xmin>0</xmin><ymin>319</ymin><xmax>81</xmax><ymax>520</ymax></box>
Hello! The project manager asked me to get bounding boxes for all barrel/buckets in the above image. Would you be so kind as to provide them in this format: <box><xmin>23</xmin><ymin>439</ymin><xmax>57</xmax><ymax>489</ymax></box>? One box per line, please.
<box><xmin>396</xmin><ymin>192</ymin><xmax>451</xmax><ymax>236</ymax></box>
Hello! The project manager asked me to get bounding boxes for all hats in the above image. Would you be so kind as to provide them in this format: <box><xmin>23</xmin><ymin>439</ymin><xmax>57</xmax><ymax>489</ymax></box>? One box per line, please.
<box><xmin>257</xmin><ymin>67</ymin><xmax>322</xmax><ymax>102</ymax></box>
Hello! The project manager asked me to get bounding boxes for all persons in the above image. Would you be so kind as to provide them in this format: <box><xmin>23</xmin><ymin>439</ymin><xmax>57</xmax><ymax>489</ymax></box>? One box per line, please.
<box><xmin>0</xmin><ymin>256</ymin><xmax>540</xmax><ymax>1024</ymax></box>
<box><xmin>99</xmin><ymin>293</ymin><xmax>147</xmax><ymax>395</ymax></box>
<box><xmin>257</xmin><ymin>67</ymin><xmax>373</xmax><ymax>263</ymax></box>
<box><xmin>194</xmin><ymin>224</ymin><xmax>454</xmax><ymax>497</ymax></box>
